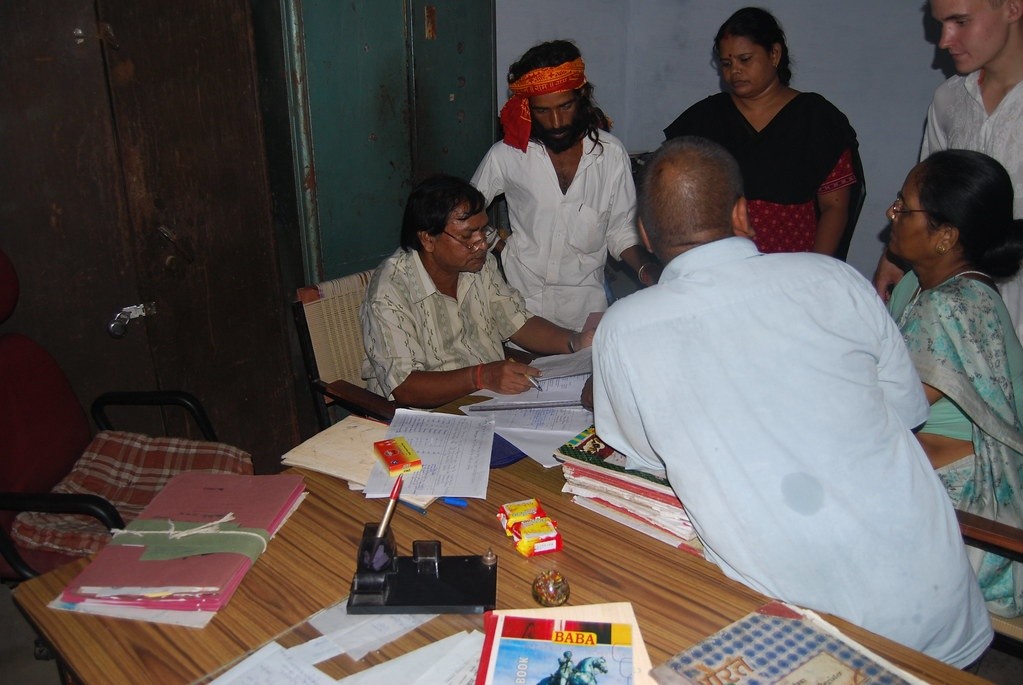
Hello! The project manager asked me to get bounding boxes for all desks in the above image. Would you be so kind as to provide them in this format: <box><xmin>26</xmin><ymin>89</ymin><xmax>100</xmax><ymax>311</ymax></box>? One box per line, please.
<box><xmin>9</xmin><ymin>466</ymin><xmax>995</xmax><ymax>685</ymax></box>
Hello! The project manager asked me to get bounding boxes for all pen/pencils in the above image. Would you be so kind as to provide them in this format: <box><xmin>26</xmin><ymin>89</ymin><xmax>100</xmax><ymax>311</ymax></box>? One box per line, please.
<box><xmin>366</xmin><ymin>472</ymin><xmax>403</xmax><ymax>569</ymax></box>
<box><xmin>397</xmin><ymin>497</ymin><xmax>426</xmax><ymax>516</ymax></box>
<box><xmin>509</xmin><ymin>358</ymin><xmax>543</xmax><ymax>391</ymax></box>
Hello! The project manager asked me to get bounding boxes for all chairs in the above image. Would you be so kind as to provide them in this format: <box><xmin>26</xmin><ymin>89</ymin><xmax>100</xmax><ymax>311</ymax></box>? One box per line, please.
<box><xmin>0</xmin><ymin>252</ymin><xmax>264</xmax><ymax>579</ymax></box>
<box><xmin>290</xmin><ymin>265</ymin><xmax>399</xmax><ymax>435</ymax></box>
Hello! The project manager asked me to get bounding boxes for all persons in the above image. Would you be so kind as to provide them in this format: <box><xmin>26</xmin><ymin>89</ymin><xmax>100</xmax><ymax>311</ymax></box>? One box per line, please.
<box><xmin>358</xmin><ymin>175</ymin><xmax>596</xmax><ymax>412</ymax></box>
<box><xmin>468</xmin><ymin>38</ymin><xmax>663</xmax><ymax>365</ymax></box>
<box><xmin>475</xmin><ymin>600</ymin><xmax>929</xmax><ymax>685</ymax></box>
<box><xmin>593</xmin><ymin>134</ymin><xmax>995</xmax><ymax>669</ymax></box>
<box><xmin>918</xmin><ymin>0</ymin><xmax>1023</xmax><ymax>346</ymax></box>
<box><xmin>663</xmin><ymin>7</ymin><xmax>859</xmax><ymax>257</ymax></box>
<box><xmin>873</xmin><ymin>147</ymin><xmax>1023</xmax><ymax>621</ymax></box>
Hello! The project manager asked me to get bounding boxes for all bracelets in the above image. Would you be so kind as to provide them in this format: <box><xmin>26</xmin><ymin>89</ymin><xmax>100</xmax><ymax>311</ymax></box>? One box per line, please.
<box><xmin>638</xmin><ymin>261</ymin><xmax>648</xmax><ymax>284</ymax></box>
<box><xmin>568</xmin><ymin>332</ymin><xmax>580</xmax><ymax>354</ymax></box>
<box><xmin>477</xmin><ymin>363</ymin><xmax>485</xmax><ymax>389</ymax></box>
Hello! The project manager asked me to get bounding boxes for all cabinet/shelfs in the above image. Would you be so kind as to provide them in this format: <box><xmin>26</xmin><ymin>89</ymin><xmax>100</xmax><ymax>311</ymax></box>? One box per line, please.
<box><xmin>259</xmin><ymin>0</ymin><xmax>496</xmax><ymax>282</ymax></box>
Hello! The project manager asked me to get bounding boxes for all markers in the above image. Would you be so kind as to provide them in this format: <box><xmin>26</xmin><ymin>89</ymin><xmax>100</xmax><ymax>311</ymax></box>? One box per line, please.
<box><xmin>439</xmin><ymin>497</ymin><xmax>468</xmax><ymax>507</ymax></box>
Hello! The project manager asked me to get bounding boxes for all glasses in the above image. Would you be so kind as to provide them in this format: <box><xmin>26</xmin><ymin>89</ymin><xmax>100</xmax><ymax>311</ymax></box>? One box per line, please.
<box><xmin>893</xmin><ymin>191</ymin><xmax>931</xmax><ymax>218</ymax></box>
<box><xmin>440</xmin><ymin>224</ymin><xmax>498</xmax><ymax>253</ymax></box>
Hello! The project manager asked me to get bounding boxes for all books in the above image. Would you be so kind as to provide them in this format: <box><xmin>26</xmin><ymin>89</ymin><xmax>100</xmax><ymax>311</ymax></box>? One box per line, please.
<box><xmin>554</xmin><ymin>424</ymin><xmax>705</xmax><ymax>558</ymax></box>
<box><xmin>61</xmin><ymin>472</ymin><xmax>306</xmax><ymax>611</ymax></box>
